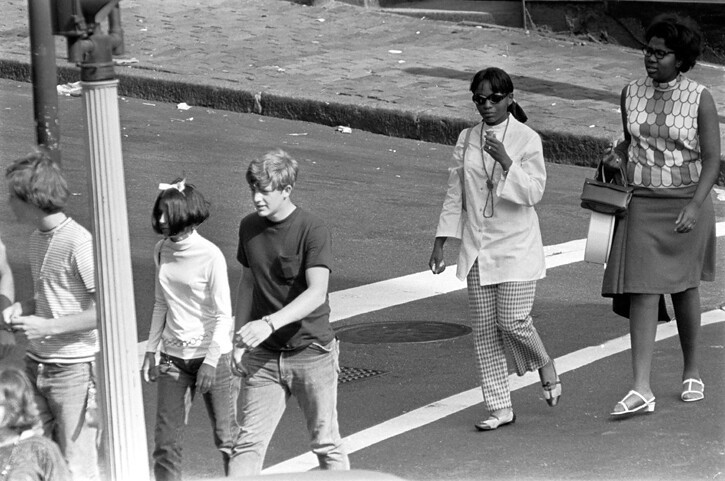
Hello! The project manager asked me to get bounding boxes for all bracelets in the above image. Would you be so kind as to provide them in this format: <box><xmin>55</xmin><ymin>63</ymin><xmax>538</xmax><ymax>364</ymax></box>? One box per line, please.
<box><xmin>503</xmin><ymin>168</ymin><xmax>511</xmax><ymax>176</ymax></box>
<box><xmin>262</xmin><ymin>316</ymin><xmax>275</xmax><ymax>333</ymax></box>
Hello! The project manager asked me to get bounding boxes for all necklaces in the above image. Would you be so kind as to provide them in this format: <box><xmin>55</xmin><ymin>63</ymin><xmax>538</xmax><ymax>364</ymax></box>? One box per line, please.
<box><xmin>480</xmin><ymin>113</ymin><xmax>509</xmax><ymax>218</ymax></box>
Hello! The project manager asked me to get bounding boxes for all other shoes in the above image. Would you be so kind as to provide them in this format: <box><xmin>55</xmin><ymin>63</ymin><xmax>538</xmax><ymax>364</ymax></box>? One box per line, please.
<box><xmin>539</xmin><ymin>359</ymin><xmax>562</xmax><ymax>407</ymax></box>
<box><xmin>475</xmin><ymin>411</ymin><xmax>516</xmax><ymax>430</ymax></box>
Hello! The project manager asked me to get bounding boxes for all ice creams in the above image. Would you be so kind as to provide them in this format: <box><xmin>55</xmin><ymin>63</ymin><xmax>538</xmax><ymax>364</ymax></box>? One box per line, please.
<box><xmin>485</xmin><ymin>129</ymin><xmax>494</xmax><ymax>137</ymax></box>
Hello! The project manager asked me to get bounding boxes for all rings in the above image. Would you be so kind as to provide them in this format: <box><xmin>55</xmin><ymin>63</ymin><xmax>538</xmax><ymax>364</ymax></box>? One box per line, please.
<box><xmin>616</xmin><ymin>158</ymin><xmax>619</xmax><ymax>162</ymax></box>
<box><xmin>488</xmin><ymin>146</ymin><xmax>492</xmax><ymax>149</ymax></box>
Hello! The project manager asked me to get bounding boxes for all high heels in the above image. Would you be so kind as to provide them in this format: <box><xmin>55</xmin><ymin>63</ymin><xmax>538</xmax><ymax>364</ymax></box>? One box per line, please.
<box><xmin>609</xmin><ymin>390</ymin><xmax>656</xmax><ymax>415</ymax></box>
<box><xmin>681</xmin><ymin>378</ymin><xmax>705</xmax><ymax>402</ymax></box>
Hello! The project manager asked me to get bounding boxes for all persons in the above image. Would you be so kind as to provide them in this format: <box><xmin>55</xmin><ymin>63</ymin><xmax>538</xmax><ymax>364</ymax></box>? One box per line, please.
<box><xmin>601</xmin><ymin>18</ymin><xmax>721</xmax><ymax>415</ymax></box>
<box><xmin>0</xmin><ymin>153</ymin><xmax>106</xmax><ymax>481</ymax></box>
<box><xmin>429</xmin><ymin>67</ymin><xmax>561</xmax><ymax>430</ymax></box>
<box><xmin>227</xmin><ymin>149</ymin><xmax>350</xmax><ymax>477</ymax></box>
<box><xmin>142</xmin><ymin>178</ymin><xmax>241</xmax><ymax>481</ymax></box>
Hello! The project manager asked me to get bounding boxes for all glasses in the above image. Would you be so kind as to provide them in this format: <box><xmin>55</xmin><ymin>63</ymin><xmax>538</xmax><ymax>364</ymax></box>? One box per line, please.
<box><xmin>472</xmin><ymin>93</ymin><xmax>509</xmax><ymax>105</ymax></box>
<box><xmin>642</xmin><ymin>46</ymin><xmax>674</xmax><ymax>59</ymax></box>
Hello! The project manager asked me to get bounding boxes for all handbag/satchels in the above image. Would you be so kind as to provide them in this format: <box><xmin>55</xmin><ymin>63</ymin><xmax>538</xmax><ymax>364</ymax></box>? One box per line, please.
<box><xmin>580</xmin><ymin>178</ymin><xmax>634</xmax><ymax>210</ymax></box>
<box><xmin>584</xmin><ymin>165</ymin><xmax>615</xmax><ymax>264</ymax></box>
